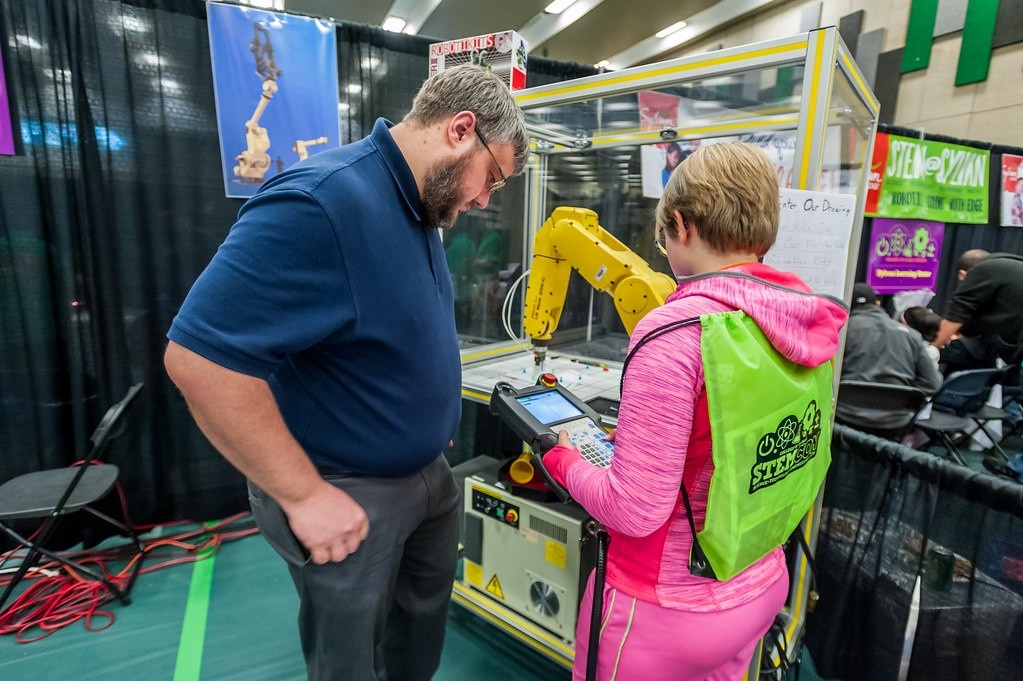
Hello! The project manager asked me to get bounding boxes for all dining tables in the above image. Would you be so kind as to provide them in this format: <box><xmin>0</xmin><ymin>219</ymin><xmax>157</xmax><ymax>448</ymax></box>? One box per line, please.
<box><xmin>813</xmin><ymin>510</ymin><xmax>1023</xmax><ymax>681</ymax></box>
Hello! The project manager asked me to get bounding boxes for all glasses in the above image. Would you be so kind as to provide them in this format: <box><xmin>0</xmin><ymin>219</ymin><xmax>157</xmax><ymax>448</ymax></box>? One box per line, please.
<box><xmin>654</xmin><ymin>218</ymin><xmax>689</xmax><ymax>258</ymax></box>
<box><xmin>472</xmin><ymin>126</ymin><xmax>507</xmax><ymax>195</ymax></box>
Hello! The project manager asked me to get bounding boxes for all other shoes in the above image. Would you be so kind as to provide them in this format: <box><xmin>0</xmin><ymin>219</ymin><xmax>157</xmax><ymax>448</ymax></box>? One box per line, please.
<box><xmin>983</xmin><ymin>456</ymin><xmax>1023</xmax><ymax>485</ymax></box>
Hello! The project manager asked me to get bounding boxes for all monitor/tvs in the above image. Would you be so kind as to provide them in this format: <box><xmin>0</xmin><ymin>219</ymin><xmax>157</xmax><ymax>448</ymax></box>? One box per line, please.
<box><xmin>516</xmin><ymin>389</ymin><xmax>585</xmax><ymax>425</ymax></box>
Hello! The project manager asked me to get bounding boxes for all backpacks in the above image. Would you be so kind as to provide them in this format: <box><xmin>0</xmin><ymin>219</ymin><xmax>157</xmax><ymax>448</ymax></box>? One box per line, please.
<box><xmin>620</xmin><ymin>311</ymin><xmax>832</xmax><ymax>582</ymax></box>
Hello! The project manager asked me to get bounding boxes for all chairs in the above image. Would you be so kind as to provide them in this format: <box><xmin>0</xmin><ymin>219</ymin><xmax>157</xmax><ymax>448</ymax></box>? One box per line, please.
<box><xmin>0</xmin><ymin>377</ymin><xmax>143</xmax><ymax>603</ymax></box>
<box><xmin>836</xmin><ymin>330</ymin><xmax>1023</xmax><ymax>466</ymax></box>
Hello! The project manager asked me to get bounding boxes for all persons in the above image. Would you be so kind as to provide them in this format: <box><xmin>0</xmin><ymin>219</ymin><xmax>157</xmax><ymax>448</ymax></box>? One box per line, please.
<box><xmin>163</xmin><ymin>63</ymin><xmax>531</xmax><ymax>681</ymax></box>
<box><xmin>541</xmin><ymin>141</ymin><xmax>850</xmax><ymax>681</ymax></box>
<box><xmin>822</xmin><ymin>247</ymin><xmax>1023</xmax><ymax>511</ymax></box>
<box><xmin>661</xmin><ymin>140</ymin><xmax>703</xmax><ymax>188</ymax></box>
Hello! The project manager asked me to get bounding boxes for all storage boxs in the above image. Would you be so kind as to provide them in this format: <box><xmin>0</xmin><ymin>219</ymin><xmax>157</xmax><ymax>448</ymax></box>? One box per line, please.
<box><xmin>428</xmin><ymin>30</ymin><xmax>528</xmax><ymax>90</ymax></box>
<box><xmin>990</xmin><ymin>535</ymin><xmax>1023</xmax><ymax>656</ymax></box>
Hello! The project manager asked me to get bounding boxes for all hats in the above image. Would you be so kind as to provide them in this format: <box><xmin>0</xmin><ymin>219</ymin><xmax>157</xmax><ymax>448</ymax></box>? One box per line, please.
<box><xmin>851</xmin><ymin>282</ymin><xmax>875</xmax><ymax>310</ymax></box>
